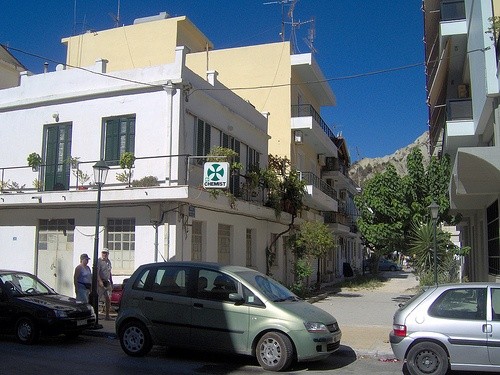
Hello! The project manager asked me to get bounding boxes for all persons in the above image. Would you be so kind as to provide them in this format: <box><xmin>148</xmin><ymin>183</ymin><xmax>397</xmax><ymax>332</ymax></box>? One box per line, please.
<box><xmin>73</xmin><ymin>254</ymin><xmax>92</xmax><ymax>304</ymax></box>
<box><xmin>96</xmin><ymin>248</ymin><xmax>117</xmax><ymax>321</ymax></box>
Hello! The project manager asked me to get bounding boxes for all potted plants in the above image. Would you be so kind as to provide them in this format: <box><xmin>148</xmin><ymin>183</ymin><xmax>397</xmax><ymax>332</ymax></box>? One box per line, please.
<box><xmin>72</xmin><ymin>169</ymin><xmax>89</xmax><ymax>189</ymax></box>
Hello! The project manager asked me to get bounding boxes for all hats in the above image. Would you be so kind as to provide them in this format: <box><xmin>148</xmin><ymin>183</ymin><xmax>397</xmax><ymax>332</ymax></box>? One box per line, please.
<box><xmin>80</xmin><ymin>253</ymin><xmax>90</xmax><ymax>260</ymax></box>
<box><xmin>101</xmin><ymin>248</ymin><xmax>109</xmax><ymax>253</ymax></box>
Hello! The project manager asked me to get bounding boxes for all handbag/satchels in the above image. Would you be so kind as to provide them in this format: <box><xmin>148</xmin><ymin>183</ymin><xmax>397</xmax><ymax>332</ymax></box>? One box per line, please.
<box><xmin>84</xmin><ymin>283</ymin><xmax>91</xmax><ymax>289</ymax></box>
<box><xmin>103</xmin><ymin>280</ymin><xmax>109</xmax><ymax>287</ymax></box>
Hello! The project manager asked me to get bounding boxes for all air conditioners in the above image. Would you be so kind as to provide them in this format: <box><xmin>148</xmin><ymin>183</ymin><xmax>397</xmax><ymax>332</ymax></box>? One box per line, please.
<box><xmin>318</xmin><ymin>153</ymin><xmax>325</xmax><ymax>165</ymax></box>
<box><xmin>458</xmin><ymin>85</ymin><xmax>467</xmax><ymax>97</ymax></box>
<box><xmin>295</xmin><ymin>130</ymin><xmax>304</xmax><ymax>145</ymax></box>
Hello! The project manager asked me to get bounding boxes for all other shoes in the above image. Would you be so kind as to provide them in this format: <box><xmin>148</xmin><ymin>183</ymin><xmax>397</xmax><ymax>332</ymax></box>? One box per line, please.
<box><xmin>105</xmin><ymin>316</ymin><xmax>115</xmax><ymax>321</ymax></box>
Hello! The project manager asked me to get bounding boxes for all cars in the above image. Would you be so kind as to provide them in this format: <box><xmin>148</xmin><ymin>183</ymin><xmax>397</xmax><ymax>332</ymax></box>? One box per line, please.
<box><xmin>0</xmin><ymin>269</ymin><xmax>97</xmax><ymax>343</ymax></box>
<box><xmin>390</xmin><ymin>282</ymin><xmax>500</xmax><ymax>375</ymax></box>
<box><xmin>115</xmin><ymin>260</ymin><xmax>344</xmax><ymax>372</ymax></box>
<box><xmin>364</xmin><ymin>257</ymin><xmax>402</xmax><ymax>272</ymax></box>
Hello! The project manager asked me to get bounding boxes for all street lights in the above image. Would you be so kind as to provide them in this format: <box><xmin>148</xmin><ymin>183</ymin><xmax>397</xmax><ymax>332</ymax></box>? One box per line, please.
<box><xmin>427</xmin><ymin>200</ymin><xmax>441</xmax><ymax>284</ymax></box>
<box><xmin>91</xmin><ymin>158</ymin><xmax>111</xmax><ymax>315</ymax></box>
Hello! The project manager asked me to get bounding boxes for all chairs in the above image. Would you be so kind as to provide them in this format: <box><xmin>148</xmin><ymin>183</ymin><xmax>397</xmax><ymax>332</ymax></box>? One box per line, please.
<box><xmin>212</xmin><ymin>276</ymin><xmax>226</xmax><ymax>298</ymax></box>
<box><xmin>198</xmin><ymin>276</ymin><xmax>209</xmax><ymax>298</ymax></box>
